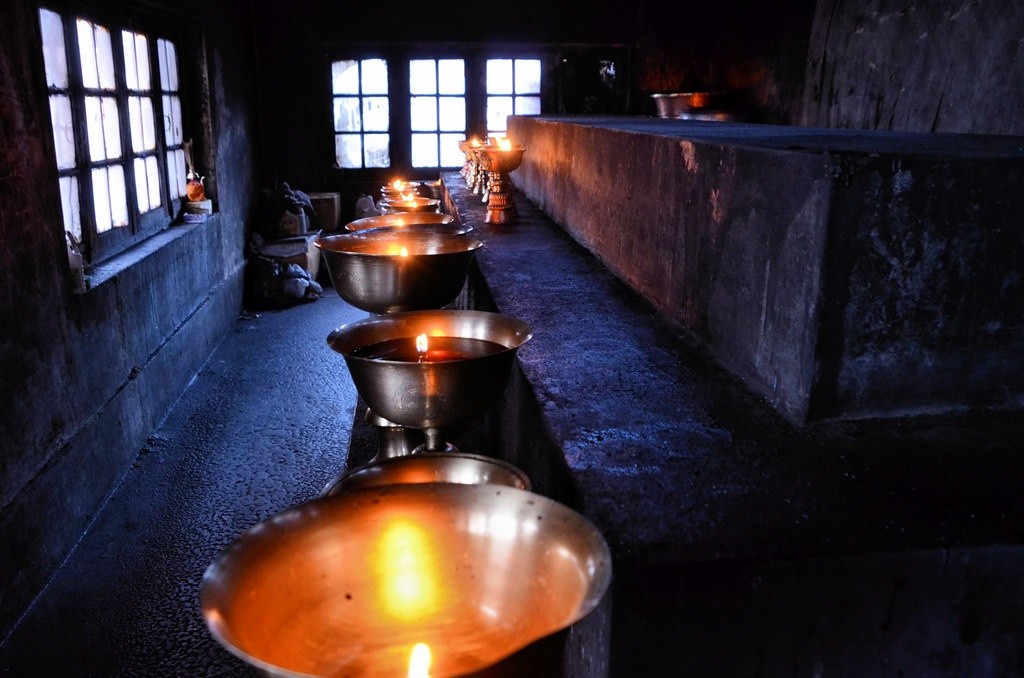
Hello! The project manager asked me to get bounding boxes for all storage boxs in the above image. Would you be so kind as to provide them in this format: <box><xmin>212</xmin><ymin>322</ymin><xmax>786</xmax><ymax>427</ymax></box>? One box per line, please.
<box><xmin>258</xmin><ymin>189</ymin><xmax>342</xmax><ymax>279</ymax></box>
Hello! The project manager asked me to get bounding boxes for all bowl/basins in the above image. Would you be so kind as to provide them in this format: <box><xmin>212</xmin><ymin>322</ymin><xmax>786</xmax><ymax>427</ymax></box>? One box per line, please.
<box><xmin>649</xmin><ymin>91</ymin><xmax>723</xmax><ymax>118</ymax></box>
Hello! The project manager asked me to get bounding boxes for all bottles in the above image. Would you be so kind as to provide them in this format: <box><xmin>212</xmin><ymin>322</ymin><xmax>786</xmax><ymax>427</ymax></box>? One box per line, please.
<box><xmin>67</xmin><ymin>244</ymin><xmax>87</xmax><ymax>294</ymax></box>
<box><xmin>298</xmin><ymin>207</ymin><xmax>308</xmax><ymax>234</ymax></box>
